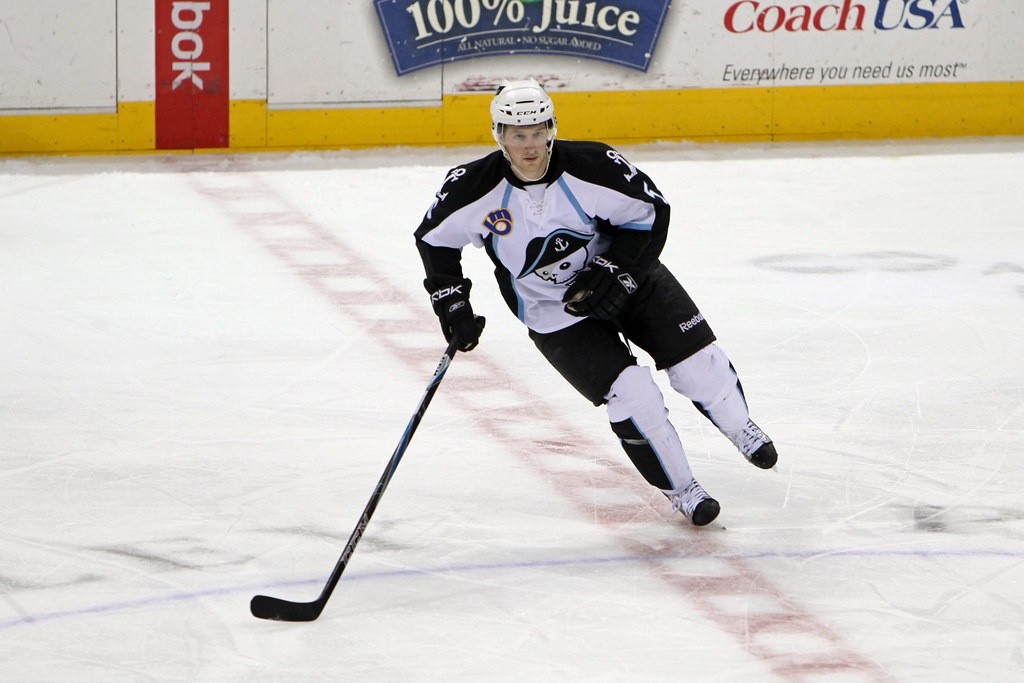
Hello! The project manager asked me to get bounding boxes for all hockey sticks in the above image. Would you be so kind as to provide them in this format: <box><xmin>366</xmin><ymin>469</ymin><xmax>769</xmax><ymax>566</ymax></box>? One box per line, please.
<box><xmin>248</xmin><ymin>314</ymin><xmax>478</xmax><ymax>623</ymax></box>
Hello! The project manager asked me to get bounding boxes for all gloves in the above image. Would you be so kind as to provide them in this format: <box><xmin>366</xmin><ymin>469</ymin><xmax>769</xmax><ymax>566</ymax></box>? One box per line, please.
<box><xmin>562</xmin><ymin>253</ymin><xmax>647</xmax><ymax>320</ymax></box>
<box><xmin>424</xmin><ymin>274</ymin><xmax>486</xmax><ymax>352</ymax></box>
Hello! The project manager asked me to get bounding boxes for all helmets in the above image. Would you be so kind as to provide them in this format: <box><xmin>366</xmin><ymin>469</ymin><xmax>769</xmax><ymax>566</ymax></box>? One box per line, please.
<box><xmin>490</xmin><ymin>77</ymin><xmax>556</xmax><ymax>146</ymax></box>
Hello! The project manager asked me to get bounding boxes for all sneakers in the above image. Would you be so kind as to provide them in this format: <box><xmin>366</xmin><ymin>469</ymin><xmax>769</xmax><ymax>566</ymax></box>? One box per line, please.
<box><xmin>662</xmin><ymin>477</ymin><xmax>727</xmax><ymax>531</ymax></box>
<box><xmin>728</xmin><ymin>417</ymin><xmax>779</xmax><ymax>474</ymax></box>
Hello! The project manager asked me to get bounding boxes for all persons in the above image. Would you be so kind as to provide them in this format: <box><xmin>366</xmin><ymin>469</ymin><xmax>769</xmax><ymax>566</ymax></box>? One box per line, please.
<box><xmin>413</xmin><ymin>76</ymin><xmax>778</xmax><ymax>531</ymax></box>
